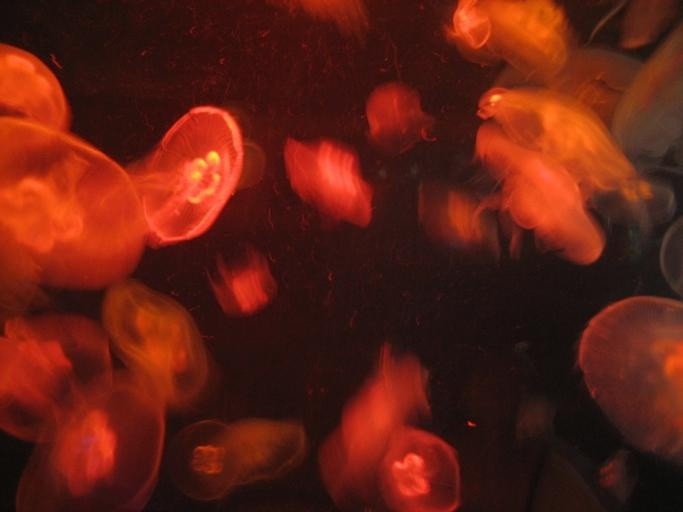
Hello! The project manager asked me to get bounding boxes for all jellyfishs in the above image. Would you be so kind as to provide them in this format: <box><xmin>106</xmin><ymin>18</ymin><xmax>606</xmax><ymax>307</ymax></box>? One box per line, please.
<box><xmin>0</xmin><ymin>0</ymin><xmax>683</xmax><ymax>512</ymax></box>
